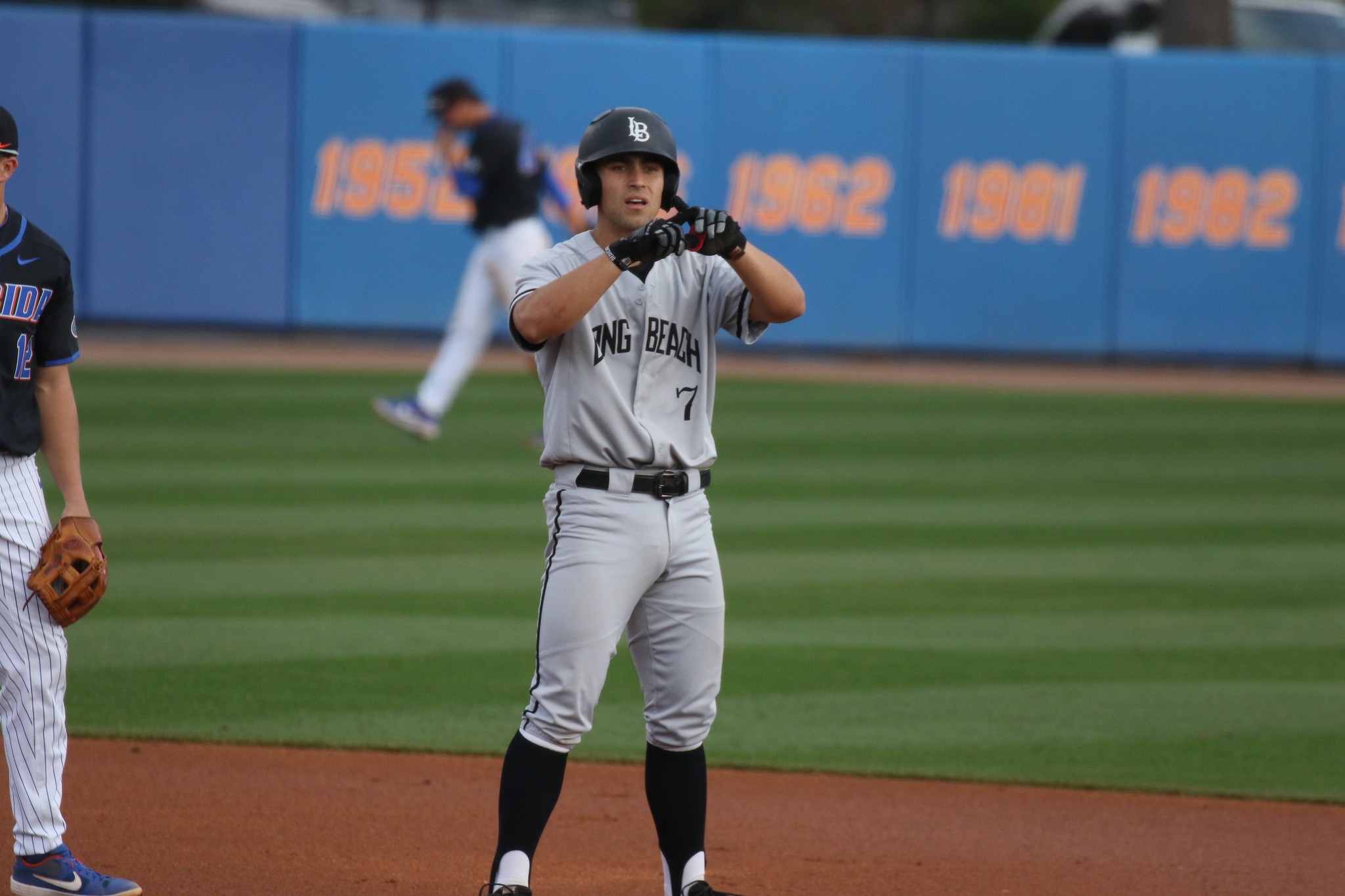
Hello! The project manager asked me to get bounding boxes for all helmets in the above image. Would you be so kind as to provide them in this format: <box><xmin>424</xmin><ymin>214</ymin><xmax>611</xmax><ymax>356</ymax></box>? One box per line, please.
<box><xmin>574</xmin><ymin>107</ymin><xmax>680</xmax><ymax>213</ymax></box>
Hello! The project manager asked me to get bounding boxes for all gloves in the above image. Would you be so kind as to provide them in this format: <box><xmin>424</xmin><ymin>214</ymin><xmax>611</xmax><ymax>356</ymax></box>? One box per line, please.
<box><xmin>665</xmin><ymin>195</ymin><xmax>747</xmax><ymax>261</ymax></box>
<box><xmin>604</xmin><ymin>206</ymin><xmax>700</xmax><ymax>271</ymax></box>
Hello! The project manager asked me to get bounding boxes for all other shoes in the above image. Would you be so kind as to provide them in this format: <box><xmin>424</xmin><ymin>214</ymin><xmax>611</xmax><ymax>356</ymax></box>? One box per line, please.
<box><xmin>369</xmin><ymin>394</ymin><xmax>439</xmax><ymax>442</ymax></box>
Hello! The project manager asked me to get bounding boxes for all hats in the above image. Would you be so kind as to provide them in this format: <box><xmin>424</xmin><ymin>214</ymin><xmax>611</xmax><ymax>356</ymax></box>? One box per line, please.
<box><xmin>428</xmin><ymin>79</ymin><xmax>472</xmax><ymax>120</ymax></box>
<box><xmin>0</xmin><ymin>106</ymin><xmax>18</xmax><ymax>158</ymax></box>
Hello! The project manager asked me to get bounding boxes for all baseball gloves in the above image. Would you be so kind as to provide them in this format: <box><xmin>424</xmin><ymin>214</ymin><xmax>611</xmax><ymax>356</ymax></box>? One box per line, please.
<box><xmin>27</xmin><ymin>517</ymin><xmax>108</xmax><ymax>629</ymax></box>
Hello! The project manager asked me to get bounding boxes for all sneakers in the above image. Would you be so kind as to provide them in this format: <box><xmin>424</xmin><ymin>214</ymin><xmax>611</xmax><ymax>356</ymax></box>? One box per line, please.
<box><xmin>682</xmin><ymin>881</ymin><xmax>744</xmax><ymax>896</ymax></box>
<box><xmin>479</xmin><ymin>883</ymin><xmax>532</xmax><ymax>896</ymax></box>
<box><xmin>10</xmin><ymin>844</ymin><xmax>142</xmax><ymax>896</ymax></box>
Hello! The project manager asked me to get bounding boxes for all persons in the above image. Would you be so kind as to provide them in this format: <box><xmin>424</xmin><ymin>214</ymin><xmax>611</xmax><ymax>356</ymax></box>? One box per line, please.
<box><xmin>0</xmin><ymin>107</ymin><xmax>143</xmax><ymax>896</ymax></box>
<box><xmin>370</xmin><ymin>79</ymin><xmax>575</xmax><ymax>444</ymax></box>
<box><xmin>476</xmin><ymin>104</ymin><xmax>809</xmax><ymax>896</ymax></box>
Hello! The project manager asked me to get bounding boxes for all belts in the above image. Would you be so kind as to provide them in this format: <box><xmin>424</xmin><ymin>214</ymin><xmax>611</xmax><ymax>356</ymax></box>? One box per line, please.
<box><xmin>576</xmin><ymin>469</ymin><xmax>711</xmax><ymax>500</ymax></box>
